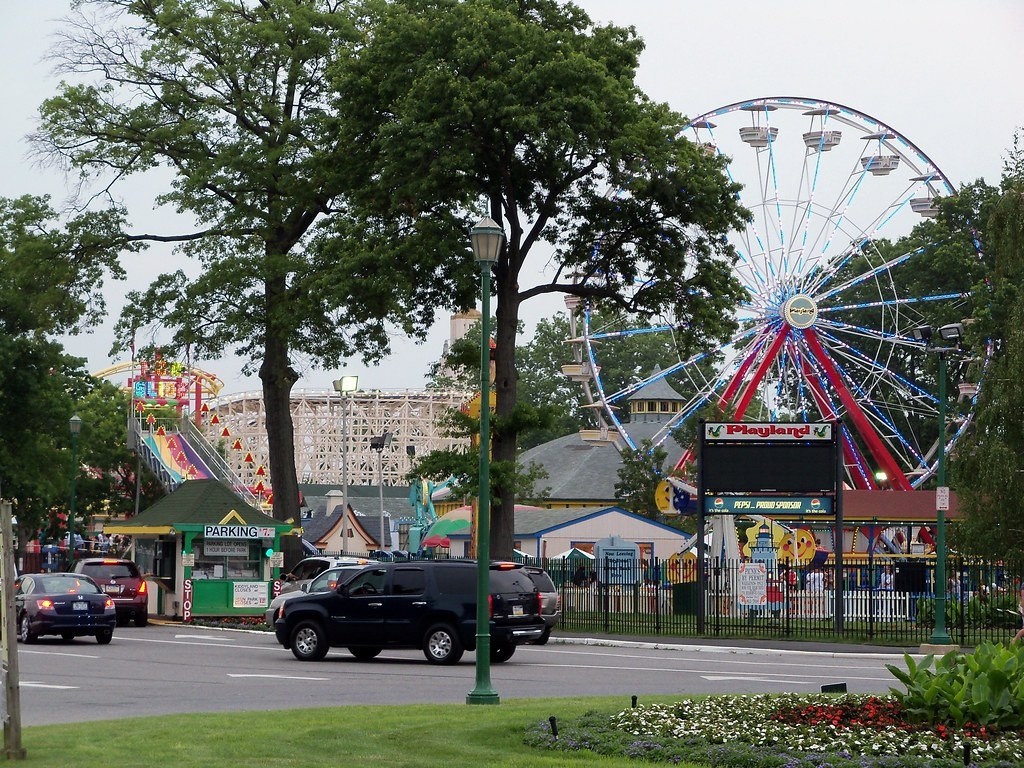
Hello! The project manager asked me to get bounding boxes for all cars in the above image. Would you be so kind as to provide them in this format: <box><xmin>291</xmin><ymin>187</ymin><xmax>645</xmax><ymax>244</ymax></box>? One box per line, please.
<box><xmin>264</xmin><ymin>567</ymin><xmax>375</xmax><ymax>630</ymax></box>
<box><xmin>275</xmin><ymin>553</ymin><xmax>386</xmax><ymax>596</ymax></box>
<box><xmin>14</xmin><ymin>573</ymin><xmax>117</xmax><ymax>645</ymax></box>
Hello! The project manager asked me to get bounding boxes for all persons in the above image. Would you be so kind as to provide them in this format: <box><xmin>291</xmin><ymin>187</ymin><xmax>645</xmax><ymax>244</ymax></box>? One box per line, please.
<box><xmin>1011</xmin><ymin>578</ymin><xmax>1024</xmax><ymax>645</ymax></box>
<box><xmin>980</xmin><ymin>582</ymin><xmax>990</xmax><ymax>604</ymax></box>
<box><xmin>779</xmin><ymin>565</ymin><xmax>834</xmax><ymax>592</ymax></box>
<box><xmin>880</xmin><ymin>564</ymin><xmax>894</xmax><ymax>590</ymax></box>
<box><xmin>61</xmin><ymin>530</ymin><xmax>130</xmax><ymax>560</ymax></box>
<box><xmin>948</xmin><ymin>570</ymin><xmax>961</xmax><ymax>594</ymax></box>
<box><xmin>892</xmin><ymin>528</ymin><xmax>904</xmax><ymax>547</ymax></box>
<box><xmin>816</xmin><ymin>538</ymin><xmax>825</xmax><ymax>550</ymax></box>
<box><xmin>286</xmin><ymin>573</ymin><xmax>300</xmax><ymax>580</ymax></box>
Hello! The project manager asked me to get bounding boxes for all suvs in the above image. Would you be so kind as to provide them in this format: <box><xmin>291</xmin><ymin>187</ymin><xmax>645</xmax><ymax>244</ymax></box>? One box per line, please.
<box><xmin>66</xmin><ymin>558</ymin><xmax>149</xmax><ymax>628</ymax></box>
<box><xmin>276</xmin><ymin>561</ymin><xmax>546</xmax><ymax>666</ymax></box>
<box><xmin>518</xmin><ymin>565</ymin><xmax>561</xmax><ymax>646</ymax></box>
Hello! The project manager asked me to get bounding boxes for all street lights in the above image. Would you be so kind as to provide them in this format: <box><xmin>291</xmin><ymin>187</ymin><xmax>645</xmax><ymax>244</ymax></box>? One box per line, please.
<box><xmin>369</xmin><ymin>432</ymin><xmax>395</xmax><ymax>554</ymax></box>
<box><xmin>331</xmin><ymin>375</ymin><xmax>360</xmax><ymax>556</ymax></box>
<box><xmin>67</xmin><ymin>413</ymin><xmax>83</xmax><ymax>570</ymax></box>
<box><xmin>465</xmin><ymin>208</ymin><xmax>503</xmax><ymax>709</ymax></box>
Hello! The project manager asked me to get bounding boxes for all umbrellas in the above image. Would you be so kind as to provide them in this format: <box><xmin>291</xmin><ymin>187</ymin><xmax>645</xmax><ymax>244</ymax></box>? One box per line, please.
<box><xmin>550</xmin><ymin>547</ymin><xmax>595</xmax><ymax>560</ymax></box>
<box><xmin>512</xmin><ymin>548</ymin><xmax>535</xmax><ymax>558</ymax></box>
